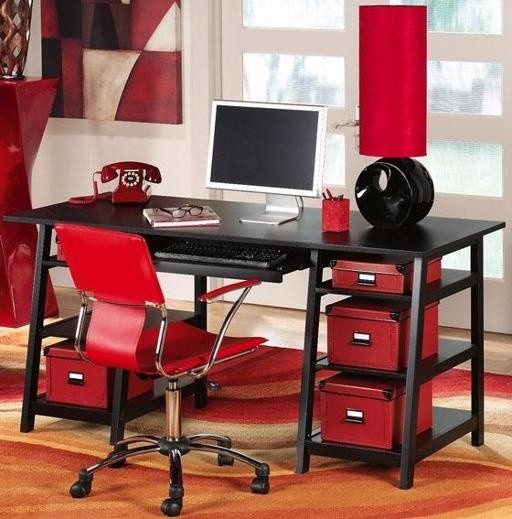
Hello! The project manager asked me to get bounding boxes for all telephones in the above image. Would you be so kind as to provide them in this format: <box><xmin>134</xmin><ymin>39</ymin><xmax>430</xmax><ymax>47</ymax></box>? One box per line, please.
<box><xmin>101</xmin><ymin>161</ymin><xmax>162</xmax><ymax>204</ymax></box>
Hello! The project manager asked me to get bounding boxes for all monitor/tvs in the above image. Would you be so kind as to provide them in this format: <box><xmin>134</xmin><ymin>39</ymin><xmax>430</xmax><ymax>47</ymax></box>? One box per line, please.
<box><xmin>204</xmin><ymin>97</ymin><xmax>328</xmax><ymax>226</ymax></box>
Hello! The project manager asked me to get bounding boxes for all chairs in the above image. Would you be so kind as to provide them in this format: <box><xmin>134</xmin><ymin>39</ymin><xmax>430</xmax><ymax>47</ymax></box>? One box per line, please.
<box><xmin>52</xmin><ymin>223</ymin><xmax>270</xmax><ymax>517</ymax></box>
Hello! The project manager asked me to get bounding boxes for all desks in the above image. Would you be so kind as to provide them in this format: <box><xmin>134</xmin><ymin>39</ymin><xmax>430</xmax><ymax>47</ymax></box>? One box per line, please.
<box><xmin>0</xmin><ymin>192</ymin><xmax>506</xmax><ymax>490</ymax></box>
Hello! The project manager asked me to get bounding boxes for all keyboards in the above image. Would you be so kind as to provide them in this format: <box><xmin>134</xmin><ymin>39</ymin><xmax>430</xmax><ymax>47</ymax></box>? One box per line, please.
<box><xmin>153</xmin><ymin>238</ymin><xmax>289</xmax><ymax>269</ymax></box>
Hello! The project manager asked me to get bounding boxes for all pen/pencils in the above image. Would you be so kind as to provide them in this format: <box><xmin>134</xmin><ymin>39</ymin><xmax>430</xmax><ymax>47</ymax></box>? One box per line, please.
<box><xmin>322</xmin><ymin>188</ymin><xmax>343</xmax><ymax>201</ymax></box>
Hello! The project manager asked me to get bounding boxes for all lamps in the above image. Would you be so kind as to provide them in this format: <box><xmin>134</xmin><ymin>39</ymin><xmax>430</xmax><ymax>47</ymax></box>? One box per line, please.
<box><xmin>355</xmin><ymin>5</ymin><xmax>435</xmax><ymax>232</ymax></box>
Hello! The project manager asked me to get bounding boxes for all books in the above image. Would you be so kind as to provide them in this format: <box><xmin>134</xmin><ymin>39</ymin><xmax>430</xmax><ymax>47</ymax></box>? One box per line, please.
<box><xmin>143</xmin><ymin>206</ymin><xmax>220</xmax><ymax>228</ymax></box>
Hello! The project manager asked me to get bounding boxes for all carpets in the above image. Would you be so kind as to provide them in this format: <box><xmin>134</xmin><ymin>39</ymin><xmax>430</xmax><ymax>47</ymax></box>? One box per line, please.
<box><xmin>1</xmin><ymin>317</ymin><xmax>512</xmax><ymax>518</ymax></box>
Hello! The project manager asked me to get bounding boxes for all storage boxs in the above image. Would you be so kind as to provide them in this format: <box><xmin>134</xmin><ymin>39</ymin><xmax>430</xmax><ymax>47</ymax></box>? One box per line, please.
<box><xmin>324</xmin><ymin>295</ymin><xmax>439</xmax><ymax>371</ymax></box>
<box><xmin>43</xmin><ymin>339</ymin><xmax>158</xmax><ymax>409</ymax></box>
<box><xmin>330</xmin><ymin>257</ymin><xmax>442</xmax><ymax>295</ymax></box>
<box><xmin>318</xmin><ymin>372</ymin><xmax>433</xmax><ymax>450</ymax></box>
